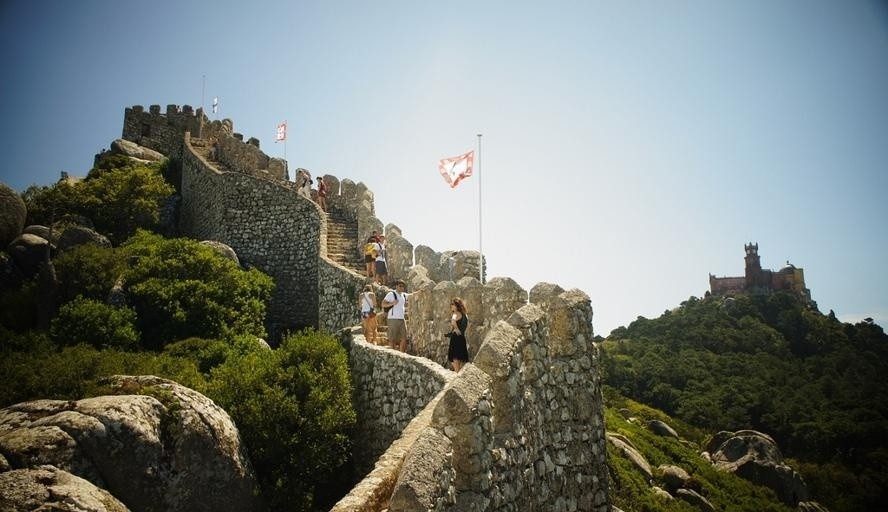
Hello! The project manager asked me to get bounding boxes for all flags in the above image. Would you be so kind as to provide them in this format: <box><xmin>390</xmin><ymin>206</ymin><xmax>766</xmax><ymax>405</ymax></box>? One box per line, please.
<box><xmin>275</xmin><ymin>122</ymin><xmax>286</xmax><ymax>144</ymax></box>
<box><xmin>437</xmin><ymin>151</ymin><xmax>474</xmax><ymax>190</ymax></box>
<box><xmin>212</xmin><ymin>97</ymin><xmax>218</xmax><ymax>114</ymax></box>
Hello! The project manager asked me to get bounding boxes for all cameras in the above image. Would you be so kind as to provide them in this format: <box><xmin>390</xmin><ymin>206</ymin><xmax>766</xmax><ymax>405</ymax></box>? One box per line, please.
<box><xmin>444</xmin><ymin>332</ymin><xmax>452</xmax><ymax>337</ymax></box>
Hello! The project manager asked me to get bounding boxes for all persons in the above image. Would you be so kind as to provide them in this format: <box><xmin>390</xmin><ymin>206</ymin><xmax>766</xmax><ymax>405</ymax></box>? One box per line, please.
<box><xmin>380</xmin><ymin>279</ymin><xmax>421</xmax><ymax>352</ymax></box>
<box><xmin>444</xmin><ymin>296</ymin><xmax>470</xmax><ymax>376</ymax></box>
<box><xmin>296</xmin><ymin>170</ymin><xmax>304</xmax><ymax>196</ymax></box>
<box><xmin>363</xmin><ymin>230</ymin><xmax>389</xmax><ymax>284</ymax></box>
<box><xmin>301</xmin><ymin>170</ymin><xmax>314</xmax><ymax>202</ymax></box>
<box><xmin>316</xmin><ymin>176</ymin><xmax>327</xmax><ymax>214</ymax></box>
<box><xmin>357</xmin><ymin>284</ymin><xmax>378</xmax><ymax>347</ymax></box>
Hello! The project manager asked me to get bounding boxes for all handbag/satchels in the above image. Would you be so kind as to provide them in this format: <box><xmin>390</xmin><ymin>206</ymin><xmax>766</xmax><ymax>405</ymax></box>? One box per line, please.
<box><xmin>369</xmin><ymin>308</ymin><xmax>376</xmax><ymax>318</ymax></box>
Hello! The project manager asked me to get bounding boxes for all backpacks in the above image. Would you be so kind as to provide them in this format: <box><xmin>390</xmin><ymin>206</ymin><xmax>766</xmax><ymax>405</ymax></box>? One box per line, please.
<box><xmin>383</xmin><ymin>291</ymin><xmax>406</xmax><ymax>316</ymax></box>
